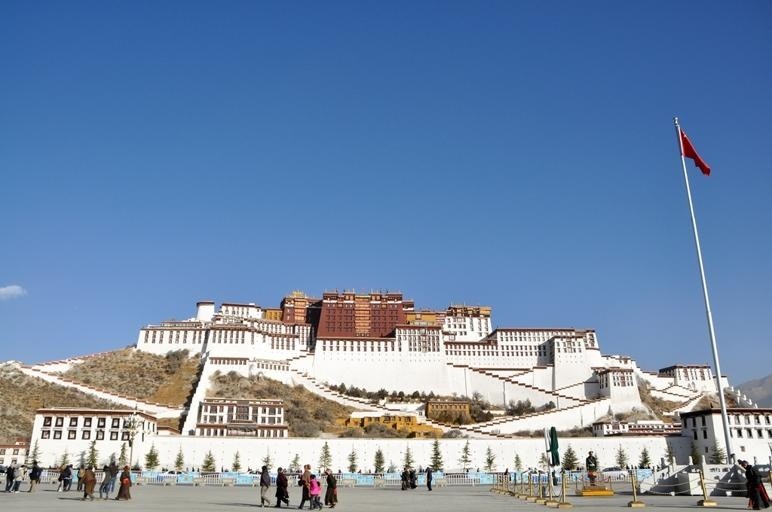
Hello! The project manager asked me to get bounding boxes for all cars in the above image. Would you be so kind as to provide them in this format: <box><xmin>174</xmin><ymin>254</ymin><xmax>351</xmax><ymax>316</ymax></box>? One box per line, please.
<box><xmin>156</xmin><ymin>468</ymin><xmax>198</xmax><ymax>485</ymax></box>
<box><xmin>751</xmin><ymin>464</ymin><xmax>771</xmax><ymax>477</ymax></box>
<box><xmin>226</xmin><ymin>469</ymin><xmax>277</xmax><ymax>487</ymax></box>
<box><xmin>600</xmin><ymin>466</ymin><xmax>629</xmax><ymax>482</ymax></box>
<box><xmin>0</xmin><ymin>464</ymin><xmax>8</xmax><ymax>474</ymax></box>
<box><xmin>521</xmin><ymin>468</ymin><xmax>561</xmax><ymax>483</ymax></box>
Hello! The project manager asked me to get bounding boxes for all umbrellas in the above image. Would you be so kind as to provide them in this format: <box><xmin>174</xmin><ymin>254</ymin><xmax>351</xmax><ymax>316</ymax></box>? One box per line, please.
<box><xmin>546</xmin><ymin>426</ymin><xmax>562</xmax><ymax>467</ymax></box>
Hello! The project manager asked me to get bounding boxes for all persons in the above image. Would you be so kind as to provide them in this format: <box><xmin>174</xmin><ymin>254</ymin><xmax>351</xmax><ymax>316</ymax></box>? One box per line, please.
<box><xmin>1</xmin><ymin>459</ymin><xmax>132</xmax><ymax>502</ymax></box>
<box><xmin>741</xmin><ymin>460</ymin><xmax>770</xmax><ymax>510</ymax></box>
<box><xmin>425</xmin><ymin>467</ymin><xmax>433</xmax><ymax>491</ymax></box>
<box><xmin>274</xmin><ymin>464</ymin><xmax>338</xmax><ymax>511</ymax></box>
<box><xmin>258</xmin><ymin>466</ymin><xmax>272</xmax><ymax>508</ymax></box>
<box><xmin>586</xmin><ymin>450</ymin><xmax>598</xmax><ymax>487</ymax></box>
<box><xmin>400</xmin><ymin>466</ymin><xmax>418</xmax><ymax>491</ymax></box>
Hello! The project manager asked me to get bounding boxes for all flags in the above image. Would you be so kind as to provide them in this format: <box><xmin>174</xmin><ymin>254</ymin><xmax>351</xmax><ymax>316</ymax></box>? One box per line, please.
<box><xmin>675</xmin><ymin>124</ymin><xmax>712</xmax><ymax>178</ymax></box>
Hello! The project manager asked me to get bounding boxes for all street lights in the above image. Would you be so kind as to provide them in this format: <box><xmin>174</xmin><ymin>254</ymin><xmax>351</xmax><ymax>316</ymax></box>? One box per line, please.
<box><xmin>127</xmin><ymin>405</ymin><xmax>140</xmax><ymax>474</ymax></box>
